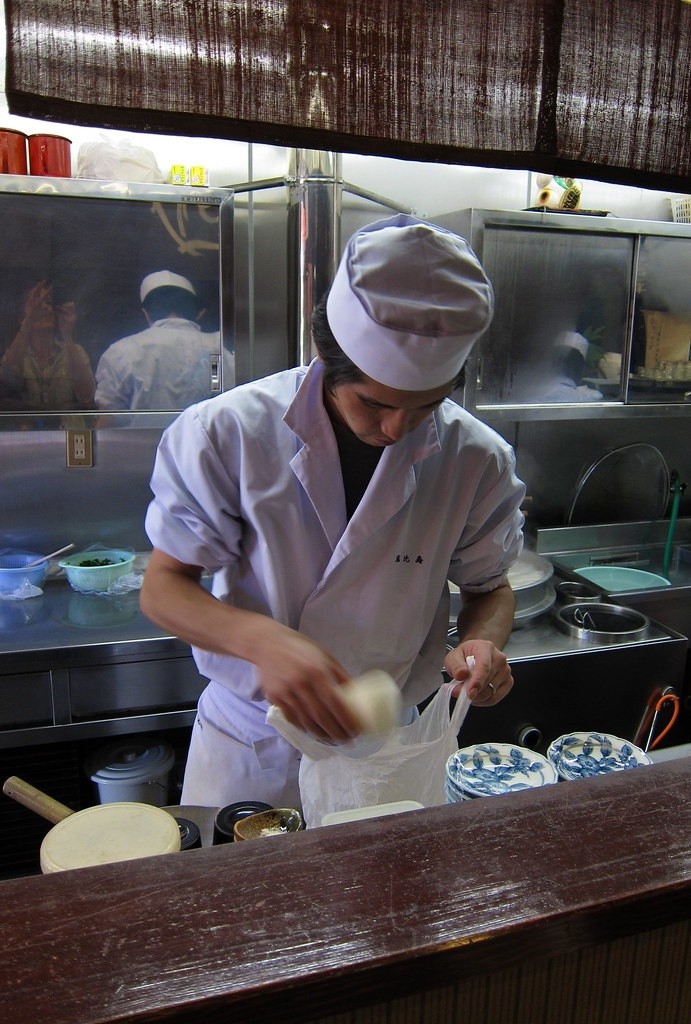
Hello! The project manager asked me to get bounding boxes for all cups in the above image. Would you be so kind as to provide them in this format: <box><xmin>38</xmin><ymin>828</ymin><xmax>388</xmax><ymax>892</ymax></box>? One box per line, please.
<box><xmin>0</xmin><ymin>127</ymin><xmax>29</xmax><ymax>176</ymax></box>
<box><xmin>174</xmin><ymin>816</ymin><xmax>203</xmax><ymax>851</ymax></box>
<box><xmin>27</xmin><ymin>133</ymin><xmax>72</xmax><ymax>178</ymax></box>
<box><xmin>213</xmin><ymin>800</ymin><xmax>274</xmax><ymax>846</ymax></box>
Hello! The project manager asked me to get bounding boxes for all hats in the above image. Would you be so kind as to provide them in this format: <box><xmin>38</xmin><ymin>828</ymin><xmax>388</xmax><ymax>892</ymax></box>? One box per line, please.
<box><xmin>553</xmin><ymin>330</ymin><xmax>591</xmax><ymax>361</ymax></box>
<box><xmin>327</xmin><ymin>212</ymin><xmax>496</xmax><ymax>392</ymax></box>
<box><xmin>140</xmin><ymin>270</ymin><xmax>196</xmax><ymax>306</ymax></box>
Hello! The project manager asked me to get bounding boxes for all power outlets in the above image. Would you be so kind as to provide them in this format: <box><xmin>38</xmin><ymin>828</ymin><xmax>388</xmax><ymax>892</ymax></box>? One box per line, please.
<box><xmin>66</xmin><ymin>431</ymin><xmax>92</xmax><ymax>467</ymax></box>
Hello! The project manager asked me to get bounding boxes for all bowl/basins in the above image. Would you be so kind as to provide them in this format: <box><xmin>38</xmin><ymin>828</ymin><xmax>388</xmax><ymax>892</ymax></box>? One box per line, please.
<box><xmin>57</xmin><ymin>549</ymin><xmax>136</xmax><ymax>592</ymax></box>
<box><xmin>0</xmin><ymin>554</ymin><xmax>49</xmax><ymax>592</ymax></box>
<box><xmin>572</xmin><ymin>566</ymin><xmax>671</xmax><ymax>592</ymax></box>
<box><xmin>60</xmin><ymin>591</ymin><xmax>140</xmax><ymax>630</ymax></box>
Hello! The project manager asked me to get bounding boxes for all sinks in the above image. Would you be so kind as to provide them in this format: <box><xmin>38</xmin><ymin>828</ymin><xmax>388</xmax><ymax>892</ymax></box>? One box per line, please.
<box><xmin>675</xmin><ymin>545</ymin><xmax>691</xmax><ymax>552</ymax></box>
<box><xmin>536</xmin><ymin>545</ymin><xmax>691</xmax><ymax>638</ymax></box>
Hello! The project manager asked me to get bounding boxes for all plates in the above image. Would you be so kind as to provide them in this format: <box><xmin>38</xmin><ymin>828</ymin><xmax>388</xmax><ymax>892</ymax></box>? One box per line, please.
<box><xmin>443</xmin><ymin>732</ymin><xmax>653</xmax><ymax>805</ymax></box>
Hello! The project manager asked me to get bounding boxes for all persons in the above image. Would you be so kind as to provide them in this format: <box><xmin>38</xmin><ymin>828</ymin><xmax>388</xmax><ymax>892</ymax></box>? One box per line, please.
<box><xmin>135</xmin><ymin>213</ymin><xmax>527</xmax><ymax>814</ymax></box>
<box><xmin>527</xmin><ymin>330</ymin><xmax>603</xmax><ymax>404</ymax></box>
<box><xmin>3</xmin><ymin>279</ymin><xmax>99</xmax><ymax>412</ymax></box>
<box><xmin>93</xmin><ymin>270</ymin><xmax>234</xmax><ymax>414</ymax></box>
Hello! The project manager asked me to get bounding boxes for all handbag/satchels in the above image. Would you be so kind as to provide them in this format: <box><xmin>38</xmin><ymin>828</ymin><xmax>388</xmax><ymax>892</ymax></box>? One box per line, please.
<box><xmin>264</xmin><ymin>652</ymin><xmax>479</xmax><ymax>830</ymax></box>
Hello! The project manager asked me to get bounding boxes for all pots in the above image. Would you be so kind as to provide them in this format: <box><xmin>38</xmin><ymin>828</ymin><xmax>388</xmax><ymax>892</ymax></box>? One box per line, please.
<box><xmin>449</xmin><ymin>550</ymin><xmax>557</xmax><ymax>633</ymax></box>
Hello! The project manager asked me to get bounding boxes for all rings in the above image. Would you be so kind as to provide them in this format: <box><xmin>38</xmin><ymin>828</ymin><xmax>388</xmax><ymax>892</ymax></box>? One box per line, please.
<box><xmin>488</xmin><ymin>682</ymin><xmax>497</xmax><ymax>695</ymax></box>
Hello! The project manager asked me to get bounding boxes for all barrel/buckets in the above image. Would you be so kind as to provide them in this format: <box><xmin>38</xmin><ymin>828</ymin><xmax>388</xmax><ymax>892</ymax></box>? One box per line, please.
<box><xmin>90</xmin><ymin>742</ymin><xmax>177</xmax><ymax>808</ymax></box>
